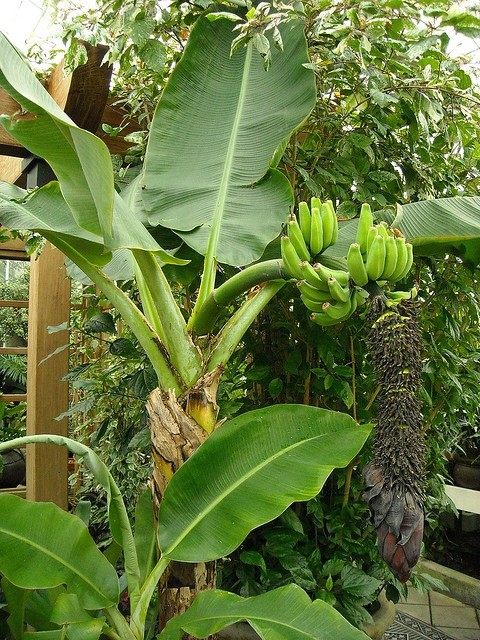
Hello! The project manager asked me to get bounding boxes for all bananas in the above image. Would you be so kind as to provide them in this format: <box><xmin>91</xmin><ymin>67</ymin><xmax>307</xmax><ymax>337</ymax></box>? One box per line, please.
<box><xmin>281</xmin><ymin>195</ymin><xmax>418</xmax><ymax>326</ymax></box>
